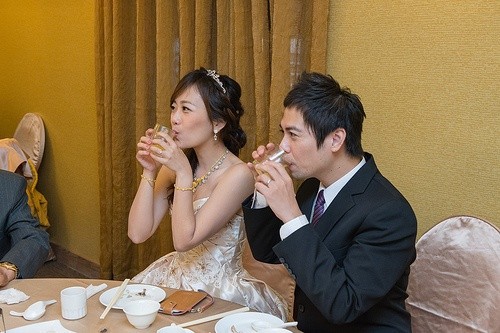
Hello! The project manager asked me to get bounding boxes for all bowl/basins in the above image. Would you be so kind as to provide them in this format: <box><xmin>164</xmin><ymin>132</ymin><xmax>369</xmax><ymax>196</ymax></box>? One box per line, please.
<box><xmin>123</xmin><ymin>301</ymin><xmax>160</xmax><ymax>329</ymax></box>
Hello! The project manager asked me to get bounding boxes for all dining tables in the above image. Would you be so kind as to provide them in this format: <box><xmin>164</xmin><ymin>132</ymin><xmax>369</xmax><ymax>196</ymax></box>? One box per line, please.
<box><xmin>0</xmin><ymin>277</ymin><xmax>305</xmax><ymax>333</ymax></box>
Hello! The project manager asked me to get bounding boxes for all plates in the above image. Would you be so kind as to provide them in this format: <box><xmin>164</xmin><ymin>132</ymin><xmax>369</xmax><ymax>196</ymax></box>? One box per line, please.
<box><xmin>214</xmin><ymin>311</ymin><xmax>286</xmax><ymax>333</ymax></box>
<box><xmin>99</xmin><ymin>284</ymin><xmax>167</xmax><ymax>310</ymax></box>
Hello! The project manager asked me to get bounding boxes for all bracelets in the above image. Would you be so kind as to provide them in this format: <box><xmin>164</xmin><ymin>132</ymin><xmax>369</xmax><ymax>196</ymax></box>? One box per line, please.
<box><xmin>141</xmin><ymin>174</ymin><xmax>156</xmax><ymax>182</ymax></box>
<box><xmin>174</xmin><ymin>183</ymin><xmax>192</xmax><ymax>191</ymax></box>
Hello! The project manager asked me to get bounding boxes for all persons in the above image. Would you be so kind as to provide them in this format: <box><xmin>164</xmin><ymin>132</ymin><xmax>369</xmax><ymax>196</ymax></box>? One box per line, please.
<box><xmin>127</xmin><ymin>66</ymin><xmax>289</xmax><ymax>324</ymax></box>
<box><xmin>0</xmin><ymin>169</ymin><xmax>49</xmax><ymax>286</ymax></box>
<box><xmin>242</xmin><ymin>69</ymin><xmax>418</xmax><ymax>333</ymax></box>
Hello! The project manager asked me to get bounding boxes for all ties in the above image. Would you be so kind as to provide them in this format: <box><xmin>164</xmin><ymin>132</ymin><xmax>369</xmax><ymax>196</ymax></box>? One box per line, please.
<box><xmin>312</xmin><ymin>189</ymin><xmax>326</xmax><ymax>225</ymax></box>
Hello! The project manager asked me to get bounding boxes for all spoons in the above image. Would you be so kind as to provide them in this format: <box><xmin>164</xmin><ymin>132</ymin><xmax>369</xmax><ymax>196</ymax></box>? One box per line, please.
<box><xmin>26</xmin><ymin>298</ymin><xmax>58</xmax><ymax>315</ymax></box>
<box><xmin>251</xmin><ymin>320</ymin><xmax>299</xmax><ymax>329</ymax></box>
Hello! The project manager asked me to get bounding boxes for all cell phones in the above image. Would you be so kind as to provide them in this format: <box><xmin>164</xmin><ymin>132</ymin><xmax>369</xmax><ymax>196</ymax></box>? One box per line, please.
<box><xmin>159</xmin><ymin>307</ymin><xmax>187</xmax><ymax>315</ymax></box>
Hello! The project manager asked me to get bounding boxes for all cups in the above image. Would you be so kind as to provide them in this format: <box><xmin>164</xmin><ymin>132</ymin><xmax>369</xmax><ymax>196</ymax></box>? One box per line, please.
<box><xmin>143</xmin><ymin>123</ymin><xmax>175</xmax><ymax>160</ymax></box>
<box><xmin>60</xmin><ymin>287</ymin><xmax>88</xmax><ymax>320</ymax></box>
<box><xmin>252</xmin><ymin>144</ymin><xmax>292</xmax><ymax>180</ymax></box>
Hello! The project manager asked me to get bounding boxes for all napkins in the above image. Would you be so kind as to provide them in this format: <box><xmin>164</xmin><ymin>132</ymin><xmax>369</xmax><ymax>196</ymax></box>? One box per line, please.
<box><xmin>0</xmin><ymin>287</ymin><xmax>31</xmax><ymax>305</ymax></box>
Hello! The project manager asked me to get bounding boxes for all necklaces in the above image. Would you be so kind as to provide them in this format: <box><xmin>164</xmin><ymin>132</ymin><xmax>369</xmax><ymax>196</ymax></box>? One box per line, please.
<box><xmin>193</xmin><ymin>148</ymin><xmax>230</xmax><ymax>192</ymax></box>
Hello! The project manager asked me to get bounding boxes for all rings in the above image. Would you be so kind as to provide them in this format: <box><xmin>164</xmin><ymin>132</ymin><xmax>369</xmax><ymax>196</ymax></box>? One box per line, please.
<box><xmin>266</xmin><ymin>179</ymin><xmax>272</xmax><ymax>184</ymax></box>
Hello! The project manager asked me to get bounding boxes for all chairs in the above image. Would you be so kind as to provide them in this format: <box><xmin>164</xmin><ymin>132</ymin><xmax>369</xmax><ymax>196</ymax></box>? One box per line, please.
<box><xmin>241</xmin><ymin>237</ymin><xmax>298</xmax><ymax>318</ymax></box>
<box><xmin>405</xmin><ymin>215</ymin><xmax>500</xmax><ymax>333</ymax></box>
<box><xmin>13</xmin><ymin>112</ymin><xmax>46</xmax><ymax>173</ymax></box>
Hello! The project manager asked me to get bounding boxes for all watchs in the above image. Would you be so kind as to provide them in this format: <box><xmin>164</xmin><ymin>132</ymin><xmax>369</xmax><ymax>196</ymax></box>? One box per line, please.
<box><xmin>0</xmin><ymin>263</ymin><xmax>16</xmax><ymax>272</ymax></box>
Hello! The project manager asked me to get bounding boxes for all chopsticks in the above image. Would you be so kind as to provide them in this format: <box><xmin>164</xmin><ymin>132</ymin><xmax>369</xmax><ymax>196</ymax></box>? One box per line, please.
<box><xmin>177</xmin><ymin>307</ymin><xmax>250</xmax><ymax>328</ymax></box>
<box><xmin>99</xmin><ymin>278</ymin><xmax>130</xmax><ymax>319</ymax></box>
